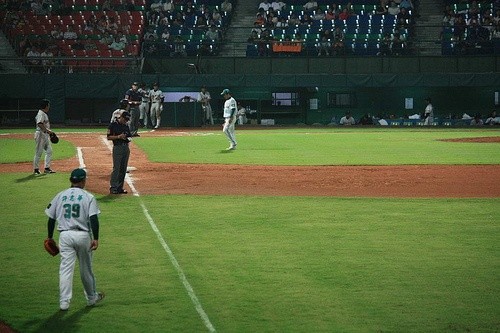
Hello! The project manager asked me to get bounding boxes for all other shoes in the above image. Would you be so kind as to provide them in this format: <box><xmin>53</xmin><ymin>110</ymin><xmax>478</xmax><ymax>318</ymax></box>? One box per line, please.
<box><xmin>226</xmin><ymin>143</ymin><xmax>237</xmax><ymax>151</ymax></box>
<box><xmin>154</xmin><ymin>125</ymin><xmax>159</xmax><ymax>129</ymax></box>
<box><xmin>131</xmin><ymin>130</ymin><xmax>138</xmax><ymax>136</ymax></box>
<box><xmin>34</xmin><ymin>169</ymin><xmax>41</xmax><ymax>175</ymax></box>
<box><xmin>44</xmin><ymin>168</ymin><xmax>57</xmax><ymax>173</ymax></box>
<box><xmin>92</xmin><ymin>292</ymin><xmax>105</xmax><ymax>305</ymax></box>
<box><xmin>110</xmin><ymin>188</ymin><xmax>128</xmax><ymax>194</ymax></box>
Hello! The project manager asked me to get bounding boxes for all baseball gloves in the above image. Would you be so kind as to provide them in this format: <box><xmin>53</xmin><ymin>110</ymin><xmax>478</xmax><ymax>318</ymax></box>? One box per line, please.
<box><xmin>49</xmin><ymin>132</ymin><xmax>58</xmax><ymax>143</ymax></box>
<box><xmin>45</xmin><ymin>239</ymin><xmax>59</xmax><ymax>256</ymax></box>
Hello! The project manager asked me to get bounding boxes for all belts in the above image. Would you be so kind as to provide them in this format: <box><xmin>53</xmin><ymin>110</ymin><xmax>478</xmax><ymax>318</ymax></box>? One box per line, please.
<box><xmin>143</xmin><ymin>101</ymin><xmax>148</xmax><ymax>103</ymax></box>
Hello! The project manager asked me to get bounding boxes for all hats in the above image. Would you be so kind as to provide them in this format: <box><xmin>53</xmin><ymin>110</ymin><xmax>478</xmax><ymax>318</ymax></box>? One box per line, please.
<box><xmin>132</xmin><ymin>82</ymin><xmax>138</xmax><ymax>85</ymax></box>
<box><xmin>220</xmin><ymin>89</ymin><xmax>230</xmax><ymax>96</ymax></box>
<box><xmin>122</xmin><ymin>112</ymin><xmax>131</xmax><ymax>121</ymax></box>
<box><xmin>49</xmin><ymin>133</ymin><xmax>59</xmax><ymax>144</ymax></box>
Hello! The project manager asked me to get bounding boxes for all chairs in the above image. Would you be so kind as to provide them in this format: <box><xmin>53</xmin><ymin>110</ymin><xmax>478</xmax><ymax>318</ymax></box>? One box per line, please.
<box><xmin>0</xmin><ymin>0</ymin><xmax>500</xmax><ymax>73</ymax></box>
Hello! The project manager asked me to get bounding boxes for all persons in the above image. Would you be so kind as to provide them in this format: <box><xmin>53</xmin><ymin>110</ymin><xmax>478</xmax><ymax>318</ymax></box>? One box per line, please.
<box><xmin>138</xmin><ymin>81</ymin><xmax>150</xmax><ymax>128</ymax></box>
<box><xmin>0</xmin><ymin>0</ymin><xmax>500</xmax><ymax>73</ymax></box>
<box><xmin>220</xmin><ymin>89</ymin><xmax>237</xmax><ymax>151</ymax></box>
<box><xmin>142</xmin><ymin>82</ymin><xmax>165</xmax><ymax>128</ymax></box>
<box><xmin>198</xmin><ymin>88</ymin><xmax>214</xmax><ymax>127</ymax></box>
<box><xmin>107</xmin><ymin>111</ymin><xmax>132</xmax><ymax>194</ymax></box>
<box><xmin>33</xmin><ymin>100</ymin><xmax>56</xmax><ymax>175</ymax></box>
<box><xmin>179</xmin><ymin>95</ymin><xmax>197</xmax><ymax>102</ymax></box>
<box><xmin>44</xmin><ymin>168</ymin><xmax>106</xmax><ymax>311</ymax></box>
<box><xmin>234</xmin><ymin>98</ymin><xmax>500</xmax><ymax>126</ymax></box>
<box><xmin>110</xmin><ymin>99</ymin><xmax>130</xmax><ymax>173</ymax></box>
<box><xmin>125</xmin><ymin>83</ymin><xmax>142</xmax><ymax>138</ymax></box>
